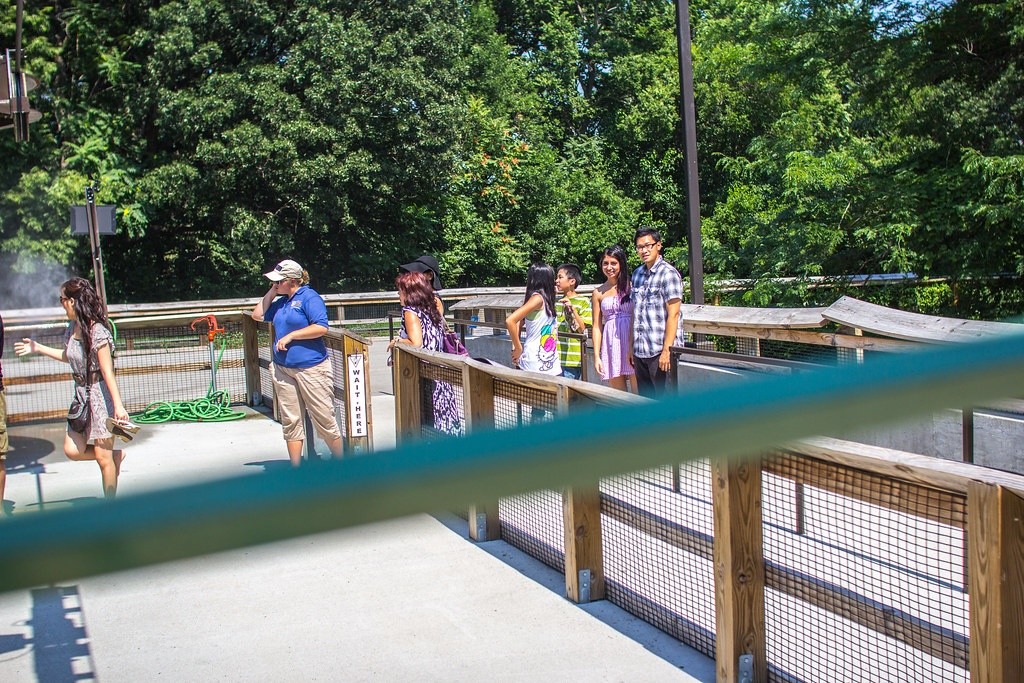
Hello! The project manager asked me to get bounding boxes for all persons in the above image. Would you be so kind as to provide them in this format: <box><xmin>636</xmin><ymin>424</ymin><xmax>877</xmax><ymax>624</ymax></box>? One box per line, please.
<box><xmin>13</xmin><ymin>274</ymin><xmax>142</xmax><ymax>501</ymax></box>
<box><xmin>385</xmin><ymin>255</ymin><xmax>469</xmax><ymax>440</ymax></box>
<box><xmin>251</xmin><ymin>258</ymin><xmax>345</xmax><ymax>466</ymax></box>
<box><xmin>591</xmin><ymin>227</ymin><xmax>685</xmax><ymax>401</ymax></box>
<box><xmin>505</xmin><ymin>262</ymin><xmax>593</xmax><ymax>423</ymax></box>
<box><xmin>0</xmin><ymin>314</ymin><xmax>9</xmax><ymax>508</ymax></box>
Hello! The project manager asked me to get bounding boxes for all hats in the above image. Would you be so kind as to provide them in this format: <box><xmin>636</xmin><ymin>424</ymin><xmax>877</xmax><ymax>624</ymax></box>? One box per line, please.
<box><xmin>399</xmin><ymin>256</ymin><xmax>442</xmax><ymax>290</ymax></box>
<box><xmin>263</xmin><ymin>260</ymin><xmax>303</xmax><ymax>281</ymax></box>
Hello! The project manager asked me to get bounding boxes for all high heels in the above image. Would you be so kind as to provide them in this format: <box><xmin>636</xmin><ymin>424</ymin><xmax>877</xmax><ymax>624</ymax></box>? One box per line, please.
<box><xmin>106</xmin><ymin>418</ymin><xmax>133</xmax><ymax>443</ymax></box>
<box><xmin>109</xmin><ymin>418</ymin><xmax>140</xmax><ymax>434</ymax></box>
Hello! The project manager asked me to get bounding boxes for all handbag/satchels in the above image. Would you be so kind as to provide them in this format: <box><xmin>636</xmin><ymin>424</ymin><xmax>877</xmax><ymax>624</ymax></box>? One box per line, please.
<box><xmin>441</xmin><ymin>316</ymin><xmax>469</xmax><ymax>356</ymax></box>
<box><xmin>67</xmin><ymin>398</ymin><xmax>90</xmax><ymax>434</ymax></box>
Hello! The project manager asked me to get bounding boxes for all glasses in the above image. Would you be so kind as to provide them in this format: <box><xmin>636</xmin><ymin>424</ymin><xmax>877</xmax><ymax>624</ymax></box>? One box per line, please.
<box><xmin>60</xmin><ymin>296</ymin><xmax>68</xmax><ymax>303</ymax></box>
<box><xmin>634</xmin><ymin>240</ymin><xmax>659</xmax><ymax>251</ymax></box>
<box><xmin>270</xmin><ymin>278</ymin><xmax>298</xmax><ymax>286</ymax></box>
